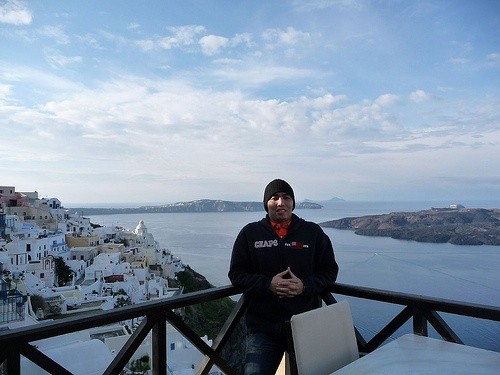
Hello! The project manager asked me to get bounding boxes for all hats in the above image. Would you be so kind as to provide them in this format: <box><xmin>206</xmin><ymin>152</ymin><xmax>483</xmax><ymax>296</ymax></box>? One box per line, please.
<box><xmin>264</xmin><ymin>178</ymin><xmax>295</xmax><ymax>214</ymax></box>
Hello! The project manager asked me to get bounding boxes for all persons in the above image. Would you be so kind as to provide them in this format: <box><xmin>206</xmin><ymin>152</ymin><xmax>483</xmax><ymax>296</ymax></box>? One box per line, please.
<box><xmin>228</xmin><ymin>179</ymin><xmax>339</xmax><ymax>375</ymax></box>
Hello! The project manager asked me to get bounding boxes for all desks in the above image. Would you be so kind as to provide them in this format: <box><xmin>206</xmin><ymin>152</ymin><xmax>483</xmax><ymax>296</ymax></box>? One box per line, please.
<box><xmin>333</xmin><ymin>333</ymin><xmax>500</xmax><ymax>375</ymax></box>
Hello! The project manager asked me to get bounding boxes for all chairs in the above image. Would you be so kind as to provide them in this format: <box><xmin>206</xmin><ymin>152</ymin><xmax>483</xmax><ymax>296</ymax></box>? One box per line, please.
<box><xmin>291</xmin><ymin>300</ymin><xmax>360</xmax><ymax>375</ymax></box>
